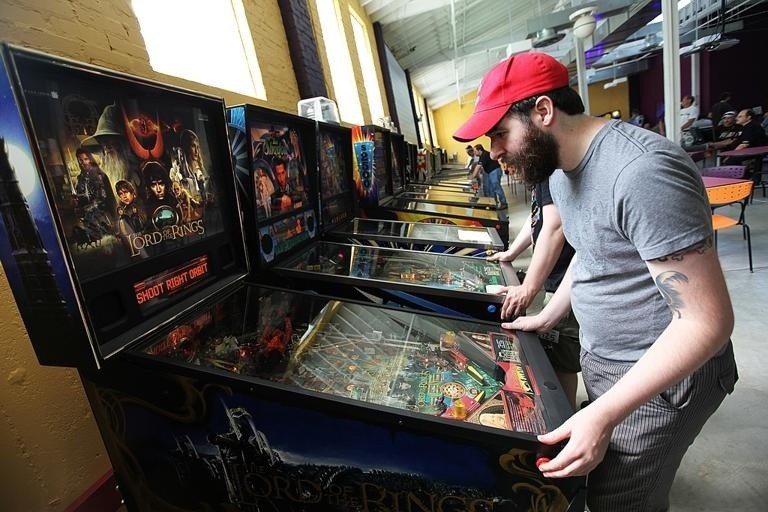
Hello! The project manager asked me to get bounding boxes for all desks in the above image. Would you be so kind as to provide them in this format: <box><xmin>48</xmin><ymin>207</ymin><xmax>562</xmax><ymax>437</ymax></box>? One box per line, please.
<box><xmin>718</xmin><ymin>146</ymin><xmax>768</xmax><ymax>201</ymax></box>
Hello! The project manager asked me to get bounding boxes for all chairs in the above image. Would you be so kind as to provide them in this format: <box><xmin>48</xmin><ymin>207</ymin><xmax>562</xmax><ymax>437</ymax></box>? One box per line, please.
<box><xmin>706</xmin><ymin>181</ymin><xmax>754</xmax><ymax>274</ymax></box>
<box><xmin>505</xmin><ymin>165</ymin><xmax>525</xmax><ymax>193</ymax></box>
<box><xmin>500</xmin><ymin>159</ymin><xmax>511</xmax><ymax>185</ymax></box>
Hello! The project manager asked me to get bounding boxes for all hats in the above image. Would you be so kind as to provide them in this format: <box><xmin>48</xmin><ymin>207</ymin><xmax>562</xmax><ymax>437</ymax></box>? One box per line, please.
<box><xmin>79</xmin><ymin>103</ymin><xmax>127</xmax><ymax>148</ymax></box>
<box><xmin>721</xmin><ymin>111</ymin><xmax>737</xmax><ymax>118</ymax></box>
<box><xmin>450</xmin><ymin>51</ymin><xmax>570</xmax><ymax>143</ymax></box>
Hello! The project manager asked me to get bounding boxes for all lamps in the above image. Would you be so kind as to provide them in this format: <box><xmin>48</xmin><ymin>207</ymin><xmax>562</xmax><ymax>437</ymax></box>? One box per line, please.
<box><xmin>569</xmin><ymin>4</ymin><xmax>598</xmax><ymax>38</ymax></box>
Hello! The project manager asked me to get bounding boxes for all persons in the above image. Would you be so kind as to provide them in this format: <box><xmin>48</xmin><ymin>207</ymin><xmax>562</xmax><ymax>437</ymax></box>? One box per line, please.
<box><xmin>116</xmin><ymin>181</ymin><xmax>150</xmax><ymax>259</ymax></box>
<box><xmin>632</xmin><ymin>109</ymin><xmax>650</xmax><ymax>128</ymax></box>
<box><xmin>761</xmin><ymin>105</ymin><xmax>768</xmax><ymax>135</ymax></box>
<box><xmin>176</xmin><ymin>146</ymin><xmax>196</xmax><ymax>179</ymax></box>
<box><xmin>142</xmin><ymin>162</ymin><xmax>177</xmax><ymax>213</ymax></box>
<box><xmin>76</xmin><ymin>145</ymin><xmax>117</xmax><ymax>234</ymax></box>
<box><xmin>713</xmin><ymin>109</ymin><xmax>768</xmax><ymax>166</ymax></box>
<box><xmin>680</xmin><ymin>95</ymin><xmax>698</xmax><ymax>146</ymax></box>
<box><xmin>181</xmin><ymin>130</ymin><xmax>209</xmax><ymax>180</ymax></box>
<box><xmin>452</xmin><ymin>51</ymin><xmax>739</xmax><ymax>512</ymax></box>
<box><xmin>81</xmin><ymin>105</ymin><xmax>141</xmax><ymax>184</ymax></box>
<box><xmin>467</xmin><ymin>144</ymin><xmax>509</xmax><ymax>210</ymax></box>
<box><xmin>466</xmin><ymin>148</ymin><xmax>479</xmax><ymax>175</ymax></box>
<box><xmin>487</xmin><ymin>178</ymin><xmax>582</xmax><ymax>414</ymax></box>
<box><xmin>178</xmin><ymin>179</ymin><xmax>182</xmax><ymax>180</ymax></box>
<box><xmin>259</xmin><ymin>174</ymin><xmax>271</xmax><ymax>219</ymax></box>
<box><xmin>710</xmin><ymin>92</ymin><xmax>733</xmax><ymax>128</ymax></box>
<box><xmin>696</xmin><ymin>113</ymin><xmax>712</xmax><ymax>127</ymax></box>
<box><xmin>270</xmin><ymin>158</ymin><xmax>302</xmax><ymax>211</ymax></box>
<box><xmin>172</xmin><ymin>181</ymin><xmax>198</xmax><ymax>222</ymax></box>
<box><xmin>715</xmin><ymin>110</ymin><xmax>743</xmax><ymax>142</ymax></box>
